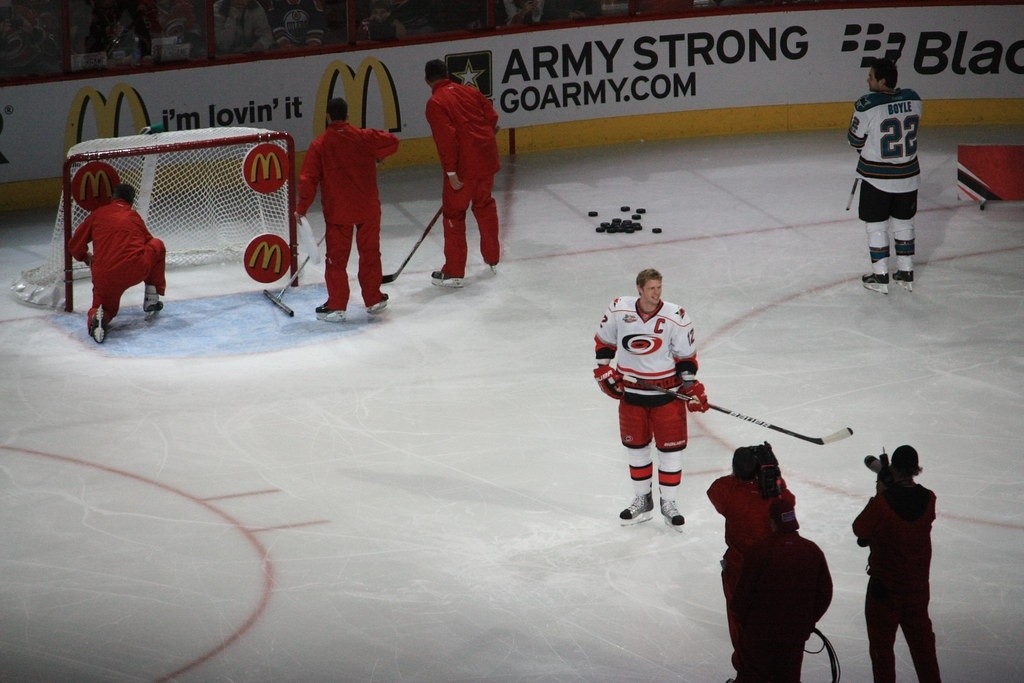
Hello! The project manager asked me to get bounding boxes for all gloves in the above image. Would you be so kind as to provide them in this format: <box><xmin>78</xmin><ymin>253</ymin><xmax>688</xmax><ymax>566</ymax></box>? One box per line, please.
<box><xmin>592</xmin><ymin>365</ymin><xmax>627</xmax><ymax>400</ymax></box>
<box><xmin>678</xmin><ymin>379</ymin><xmax>710</xmax><ymax>414</ymax></box>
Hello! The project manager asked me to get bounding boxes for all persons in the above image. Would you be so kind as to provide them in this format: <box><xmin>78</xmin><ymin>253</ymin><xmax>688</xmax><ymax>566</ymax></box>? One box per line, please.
<box><xmin>69</xmin><ymin>183</ymin><xmax>166</xmax><ymax>344</ymax></box>
<box><xmin>854</xmin><ymin>445</ymin><xmax>942</xmax><ymax>683</ymax></box>
<box><xmin>295</xmin><ymin>98</ymin><xmax>401</xmax><ymax>320</ymax></box>
<box><xmin>848</xmin><ymin>59</ymin><xmax>921</xmax><ymax>294</ymax></box>
<box><xmin>593</xmin><ymin>268</ymin><xmax>708</xmax><ymax>533</ymax></box>
<box><xmin>707</xmin><ymin>444</ymin><xmax>833</xmax><ymax>683</ymax></box>
<box><xmin>424</xmin><ymin>59</ymin><xmax>500</xmax><ymax>288</ymax></box>
<box><xmin>1</xmin><ymin>0</ymin><xmax>777</xmax><ymax>73</ymax></box>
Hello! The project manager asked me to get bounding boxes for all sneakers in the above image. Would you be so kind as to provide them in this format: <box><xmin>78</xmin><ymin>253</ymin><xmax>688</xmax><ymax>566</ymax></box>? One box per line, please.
<box><xmin>315</xmin><ymin>296</ymin><xmax>346</xmax><ymax>322</ymax></box>
<box><xmin>863</xmin><ymin>272</ymin><xmax>889</xmax><ymax>295</ymax></box>
<box><xmin>619</xmin><ymin>486</ymin><xmax>654</xmax><ymax>525</ymax></box>
<box><xmin>488</xmin><ymin>262</ymin><xmax>496</xmax><ymax>275</ymax></box>
<box><xmin>143</xmin><ymin>284</ymin><xmax>163</xmax><ymax>324</ymax></box>
<box><xmin>892</xmin><ymin>268</ymin><xmax>913</xmax><ymax>293</ymax></box>
<box><xmin>432</xmin><ymin>269</ymin><xmax>465</xmax><ymax>287</ymax></box>
<box><xmin>90</xmin><ymin>303</ymin><xmax>106</xmax><ymax>344</ymax></box>
<box><xmin>366</xmin><ymin>291</ymin><xmax>388</xmax><ymax>320</ymax></box>
<box><xmin>659</xmin><ymin>487</ymin><xmax>684</xmax><ymax>532</ymax></box>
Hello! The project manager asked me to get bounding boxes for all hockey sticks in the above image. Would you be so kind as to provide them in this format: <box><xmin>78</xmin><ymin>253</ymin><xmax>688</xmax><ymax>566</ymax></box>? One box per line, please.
<box><xmin>381</xmin><ymin>125</ymin><xmax>500</xmax><ymax>284</ymax></box>
<box><xmin>846</xmin><ymin>178</ymin><xmax>859</xmax><ymax>210</ymax></box>
<box><xmin>621</xmin><ymin>375</ymin><xmax>853</xmax><ymax>445</ymax></box>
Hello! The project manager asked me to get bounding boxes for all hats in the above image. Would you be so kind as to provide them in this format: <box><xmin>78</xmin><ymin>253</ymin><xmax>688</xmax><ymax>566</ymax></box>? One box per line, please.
<box><xmin>892</xmin><ymin>444</ymin><xmax>918</xmax><ymax>470</ymax></box>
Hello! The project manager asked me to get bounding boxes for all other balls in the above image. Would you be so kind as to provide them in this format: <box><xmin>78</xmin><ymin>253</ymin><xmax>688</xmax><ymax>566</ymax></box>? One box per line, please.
<box><xmin>652</xmin><ymin>228</ymin><xmax>662</xmax><ymax>233</ymax></box>
<box><xmin>637</xmin><ymin>209</ymin><xmax>645</xmax><ymax>213</ymax></box>
<box><xmin>596</xmin><ymin>218</ymin><xmax>642</xmax><ymax>233</ymax></box>
<box><xmin>589</xmin><ymin>212</ymin><xmax>598</xmax><ymax>216</ymax></box>
<box><xmin>621</xmin><ymin>207</ymin><xmax>630</xmax><ymax>211</ymax></box>
<box><xmin>632</xmin><ymin>215</ymin><xmax>641</xmax><ymax>219</ymax></box>
